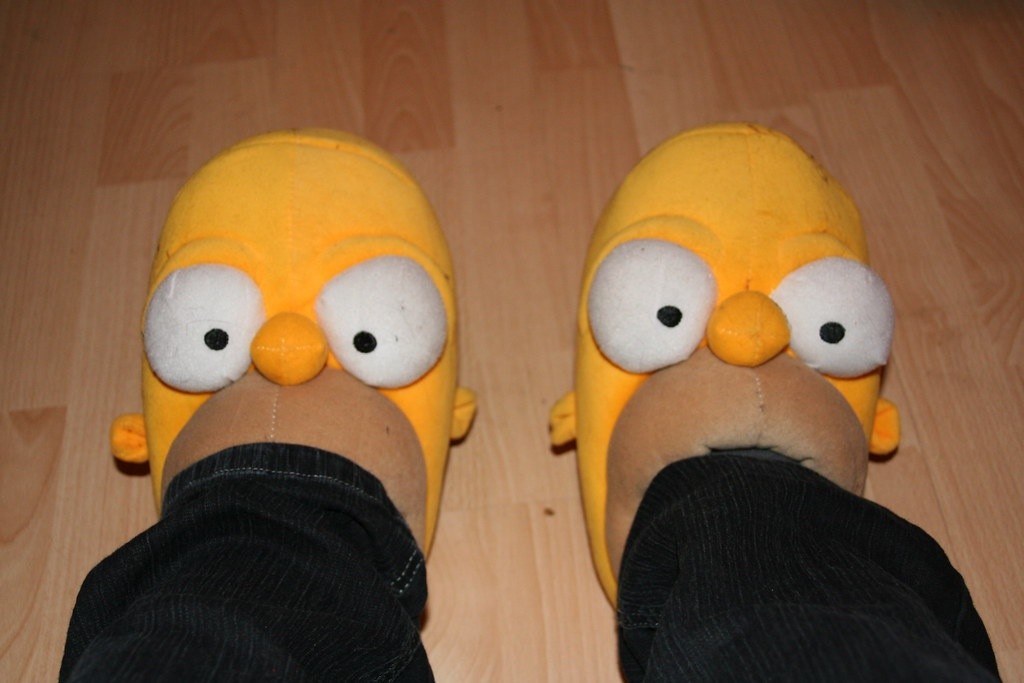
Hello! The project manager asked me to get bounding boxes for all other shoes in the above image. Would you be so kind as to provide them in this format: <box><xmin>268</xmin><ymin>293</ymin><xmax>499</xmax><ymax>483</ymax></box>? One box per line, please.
<box><xmin>112</xmin><ymin>128</ymin><xmax>475</xmax><ymax>637</ymax></box>
<box><xmin>547</xmin><ymin>124</ymin><xmax>900</xmax><ymax>616</ymax></box>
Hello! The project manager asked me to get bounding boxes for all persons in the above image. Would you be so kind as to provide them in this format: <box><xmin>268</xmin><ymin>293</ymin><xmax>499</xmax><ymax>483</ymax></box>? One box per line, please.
<box><xmin>58</xmin><ymin>125</ymin><xmax>1004</xmax><ymax>682</ymax></box>
